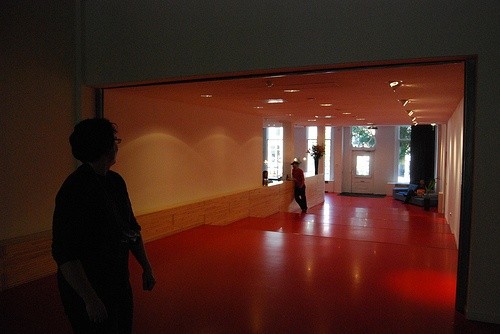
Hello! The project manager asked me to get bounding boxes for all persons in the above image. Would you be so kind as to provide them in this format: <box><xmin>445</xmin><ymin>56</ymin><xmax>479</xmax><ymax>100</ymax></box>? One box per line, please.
<box><xmin>291</xmin><ymin>162</ymin><xmax>308</xmax><ymax>214</ymax></box>
<box><xmin>52</xmin><ymin>118</ymin><xmax>156</xmax><ymax>334</ymax></box>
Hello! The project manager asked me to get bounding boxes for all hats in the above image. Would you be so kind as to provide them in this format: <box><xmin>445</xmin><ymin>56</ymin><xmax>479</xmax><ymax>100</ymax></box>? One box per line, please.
<box><xmin>290</xmin><ymin>157</ymin><xmax>300</xmax><ymax>166</ymax></box>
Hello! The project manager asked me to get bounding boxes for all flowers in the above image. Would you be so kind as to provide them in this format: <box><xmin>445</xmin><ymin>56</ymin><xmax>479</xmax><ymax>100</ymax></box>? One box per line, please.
<box><xmin>311</xmin><ymin>143</ymin><xmax>324</xmax><ymax>158</ymax></box>
<box><xmin>419</xmin><ymin>176</ymin><xmax>440</xmax><ymax>195</ymax></box>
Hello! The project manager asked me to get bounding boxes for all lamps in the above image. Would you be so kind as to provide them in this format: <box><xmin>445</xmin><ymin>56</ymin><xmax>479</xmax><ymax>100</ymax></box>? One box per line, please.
<box><xmin>401</xmin><ymin>99</ymin><xmax>409</xmax><ymax>106</ymax></box>
<box><xmin>405</xmin><ymin>110</ymin><xmax>418</xmax><ymax>126</ymax></box>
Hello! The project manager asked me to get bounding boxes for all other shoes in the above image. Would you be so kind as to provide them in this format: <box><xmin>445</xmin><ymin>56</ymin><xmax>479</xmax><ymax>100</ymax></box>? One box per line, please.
<box><xmin>302</xmin><ymin>208</ymin><xmax>307</xmax><ymax>213</ymax></box>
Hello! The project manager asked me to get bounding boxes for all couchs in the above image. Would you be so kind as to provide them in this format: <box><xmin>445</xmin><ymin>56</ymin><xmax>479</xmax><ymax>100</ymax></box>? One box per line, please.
<box><xmin>393</xmin><ymin>187</ymin><xmax>437</xmax><ymax>207</ymax></box>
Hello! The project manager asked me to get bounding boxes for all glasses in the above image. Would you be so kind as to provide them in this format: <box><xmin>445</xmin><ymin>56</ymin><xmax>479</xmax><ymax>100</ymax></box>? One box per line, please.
<box><xmin>114</xmin><ymin>138</ymin><xmax>121</xmax><ymax>145</ymax></box>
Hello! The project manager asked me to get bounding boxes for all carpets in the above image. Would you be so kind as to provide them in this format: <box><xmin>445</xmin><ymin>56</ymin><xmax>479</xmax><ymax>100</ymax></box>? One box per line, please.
<box><xmin>337</xmin><ymin>192</ymin><xmax>387</xmax><ymax>198</ymax></box>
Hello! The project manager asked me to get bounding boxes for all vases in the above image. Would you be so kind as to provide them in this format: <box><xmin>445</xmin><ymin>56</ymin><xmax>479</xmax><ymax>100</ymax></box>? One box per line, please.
<box><xmin>314</xmin><ymin>155</ymin><xmax>318</xmax><ymax>175</ymax></box>
<box><xmin>424</xmin><ymin>196</ymin><xmax>430</xmax><ymax>212</ymax></box>
